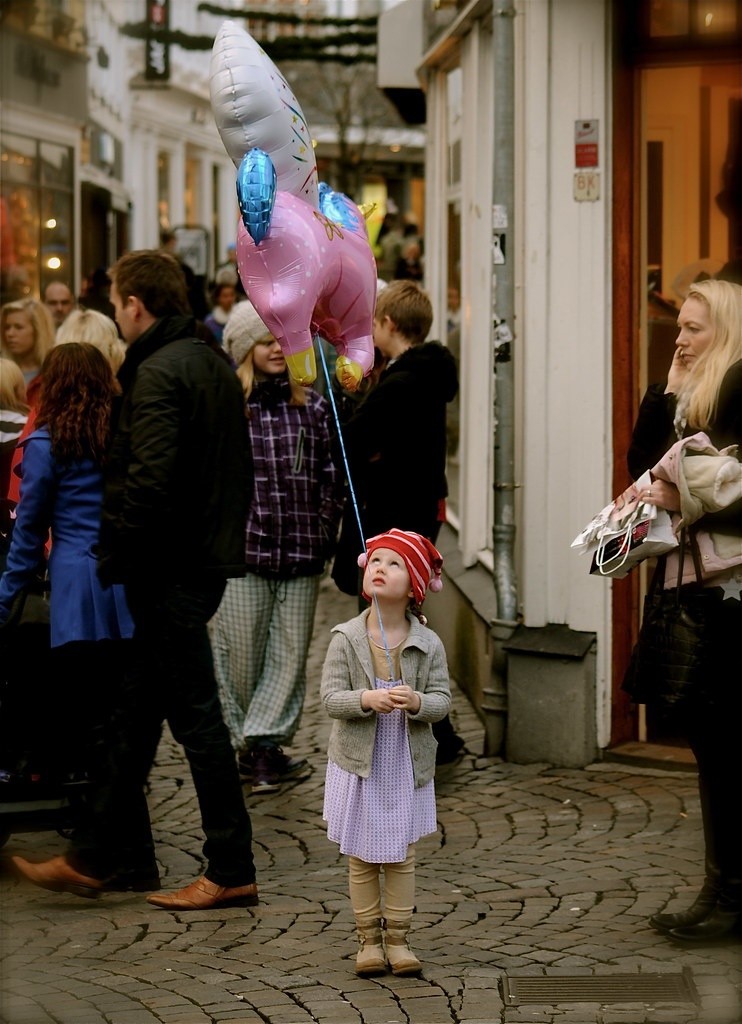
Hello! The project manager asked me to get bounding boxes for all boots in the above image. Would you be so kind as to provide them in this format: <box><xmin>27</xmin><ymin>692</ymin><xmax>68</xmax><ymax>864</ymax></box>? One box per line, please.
<box><xmin>649</xmin><ymin>856</ymin><xmax>742</xmax><ymax>941</ymax></box>
<box><xmin>384</xmin><ymin>916</ymin><xmax>422</xmax><ymax>975</ymax></box>
<box><xmin>354</xmin><ymin>920</ymin><xmax>386</xmax><ymax>973</ymax></box>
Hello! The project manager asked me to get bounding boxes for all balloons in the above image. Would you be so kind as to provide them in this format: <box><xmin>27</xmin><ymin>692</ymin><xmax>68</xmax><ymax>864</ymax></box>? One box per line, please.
<box><xmin>211</xmin><ymin>19</ymin><xmax>377</xmax><ymax>391</ymax></box>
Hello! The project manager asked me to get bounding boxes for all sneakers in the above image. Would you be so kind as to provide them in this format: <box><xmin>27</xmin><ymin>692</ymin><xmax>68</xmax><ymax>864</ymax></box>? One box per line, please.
<box><xmin>248</xmin><ymin>737</ymin><xmax>286</xmax><ymax>792</ymax></box>
<box><xmin>237</xmin><ymin>748</ymin><xmax>311</xmax><ymax>781</ymax></box>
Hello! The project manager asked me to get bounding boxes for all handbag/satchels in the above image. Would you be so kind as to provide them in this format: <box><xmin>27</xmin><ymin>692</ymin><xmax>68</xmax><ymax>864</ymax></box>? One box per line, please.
<box><xmin>620</xmin><ymin>528</ymin><xmax>731</xmax><ymax>714</ymax></box>
<box><xmin>569</xmin><ymin>469</ymin><xmax>680</xmax><ymax>579</ymax></box>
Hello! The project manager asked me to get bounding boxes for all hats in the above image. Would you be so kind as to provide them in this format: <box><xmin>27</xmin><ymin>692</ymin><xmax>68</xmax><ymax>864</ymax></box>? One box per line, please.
<box><xmin>220</xmin><ymin>298</ymin><xmax>269</xmax><ymax>368</ymax></box>
<box><xmin>356</xmin><ymin>528</ymin><xmax>444</xmax><ymax>627</ymax></box>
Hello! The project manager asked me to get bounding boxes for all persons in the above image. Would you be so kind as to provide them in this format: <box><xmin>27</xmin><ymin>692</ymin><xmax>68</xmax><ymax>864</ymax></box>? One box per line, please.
<box><xmin>0</xmin><ymin>213</ymin><xmax>464</xmax><ymax>788</ymax></box>
<box><xmin>10</xmin><ymin>249</ymin><xmax>257</xmax><ymax>911</ymax></box>
<box><xmin>626</xmin><ymin>280</ymin><xmax>742</xmax><ymax>948</ymax></box>
<box><xmin>320</xmin><ymin>529</ymin><xmax>451</xmax><ymax>972</ymax></box>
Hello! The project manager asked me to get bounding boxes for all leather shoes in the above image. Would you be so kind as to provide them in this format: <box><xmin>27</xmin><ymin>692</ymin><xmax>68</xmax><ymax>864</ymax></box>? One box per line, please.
<box><xmin>11</xmin><ymin>855</ymin><xmax>101</xmax><ymax>899</ymax></box>
<box><xmin>145</xmin><ymin>873</ymin><xmax>260</xmax><ymax>910</ymax></box>
